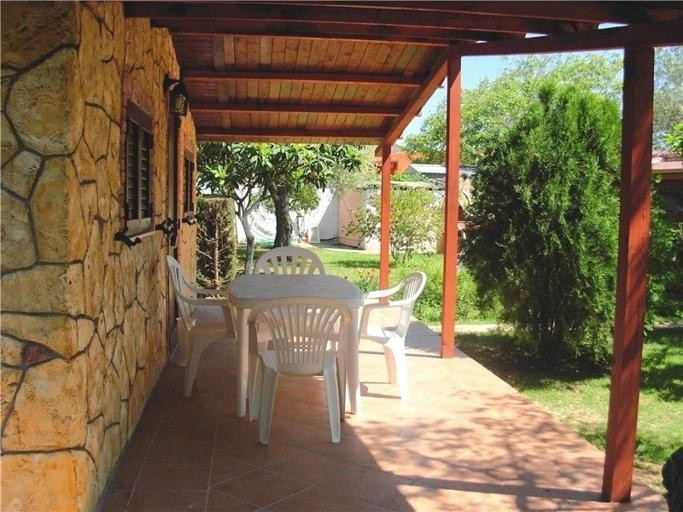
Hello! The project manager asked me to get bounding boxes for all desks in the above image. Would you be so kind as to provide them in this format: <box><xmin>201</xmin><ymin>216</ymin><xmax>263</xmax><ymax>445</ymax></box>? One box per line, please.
<box><xmin>227</xmin><ymin>275</ymin><xmax>363</xmax><ymax>416</ymax></box>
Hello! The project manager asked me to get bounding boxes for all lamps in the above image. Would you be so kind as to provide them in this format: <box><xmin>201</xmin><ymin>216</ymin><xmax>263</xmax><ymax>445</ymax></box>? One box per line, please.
<box><xmin>165</xmin><ymin>74</ymin><xmax>190</xmax><ymax>116</ymax></box>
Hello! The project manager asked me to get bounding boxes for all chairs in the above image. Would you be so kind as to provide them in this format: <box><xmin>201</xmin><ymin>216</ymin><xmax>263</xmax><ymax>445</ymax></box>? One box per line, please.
<box><xmin>254</xmin><ymin>246</ymin><xmax>325</xmax><ymax>274</ymax></box>
<box><xmin>167</xmin><ymin>254</ymin><xmax>238</xmax><ymax>395</ymax></box>
<box><xmin>357</xmin><ymin>270</ymin><xmax>427</xmax><ymax>402</ymax></box>
<box><xmin>246</xmin><ymin>299</ymin><xmax>348</xmax><ymax>443</ymax></box>
